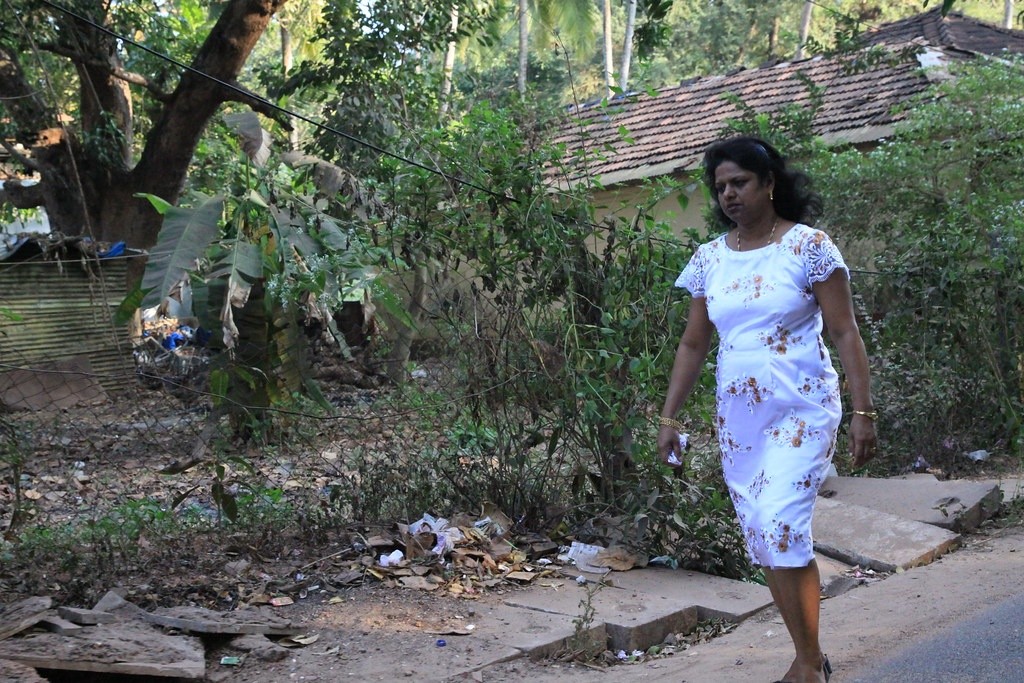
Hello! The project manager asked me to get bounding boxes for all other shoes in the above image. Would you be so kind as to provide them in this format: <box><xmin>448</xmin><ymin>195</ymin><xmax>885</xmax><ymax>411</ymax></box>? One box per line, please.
<box><xmin>773</xmin><ymin>653</ymin><xmax>832</xmax><ymax>683</ymax></box>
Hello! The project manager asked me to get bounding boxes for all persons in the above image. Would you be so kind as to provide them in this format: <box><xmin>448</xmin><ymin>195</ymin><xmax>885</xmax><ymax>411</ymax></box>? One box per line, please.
<box><xmin>656</xmin><ymin>136</ymin><xmax>877</xmax><ymax>682</ymax></box>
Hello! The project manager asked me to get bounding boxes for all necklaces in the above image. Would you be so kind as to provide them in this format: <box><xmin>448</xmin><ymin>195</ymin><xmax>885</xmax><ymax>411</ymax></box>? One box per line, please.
<box><xmin>736</xmin><ymin>215</ymin><xmax>778</xmax><ymax>252</ymax></box>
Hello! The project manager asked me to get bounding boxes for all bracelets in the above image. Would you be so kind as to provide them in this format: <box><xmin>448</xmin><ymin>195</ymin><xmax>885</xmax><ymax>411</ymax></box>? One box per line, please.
<box><xmin>852</xmin><ymin>410</ymin><xmax>878</xmax><ymax>420</ymax></box>
<box><xmin>658</xmin><ymin>417</ymin><xmax>681</xmax><ymax>431</ymax></box>
